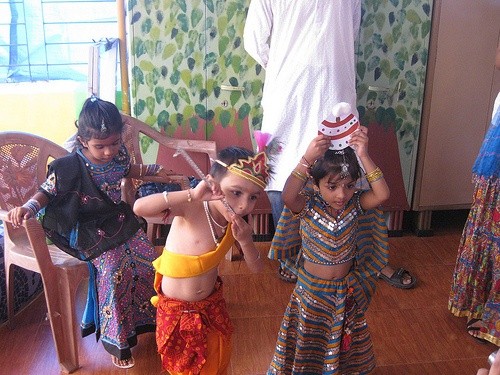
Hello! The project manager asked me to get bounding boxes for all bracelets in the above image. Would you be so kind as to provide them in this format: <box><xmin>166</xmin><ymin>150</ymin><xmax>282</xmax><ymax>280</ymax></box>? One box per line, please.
<box><xmin>290</xmin><ymin>157</ymin><xmax>312</xmax><ymax>183</ymax></box>
<box><xmin>23</xmin><ymin>199</ymin><xmax>41</xmax><ymax>216</ymax></box>
<box><xmin>244</xmin><ymin>250</ymin><xmax>261</xmax><ymax>263</ymax></box>
<box><xmin>187</xmin><ymin>188</ymin><xmax>192</xmax><ymax>203</ymax></box>
<box><xmin>365</xmin><ymin>167</ymin><xmax>383</xmax><ymax>183</ymax></box>
<box><xmin>163</xmin><ymin>191</ymin><xmax>172</xmax><ymax>210</ymax></box>
<box><xmin>139</xmin><ymin>164</ymin><xmax>163</xmax><ymax>177</ymax></box>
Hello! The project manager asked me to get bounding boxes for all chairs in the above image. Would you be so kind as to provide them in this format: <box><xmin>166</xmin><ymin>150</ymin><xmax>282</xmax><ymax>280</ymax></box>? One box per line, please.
<box><xmin>121</xmin><ymin>112</ymin><xmax>241</xmax><ymax>263</ymax></box>
<box><xmin>0</xmin><ymin>131</ymin><xmax>135</xmax><ymax>375</ymax></box>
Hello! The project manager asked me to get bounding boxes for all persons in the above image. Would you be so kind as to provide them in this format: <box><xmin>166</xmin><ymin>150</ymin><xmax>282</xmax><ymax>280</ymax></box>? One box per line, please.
<box><xmin>243</xmin><ymin>0</ymin><xmax>417</xmax><ymax>289</ymax></box>
<box><xmin>8</xmin><ymin>95</ymin><xmax>170</xmax><ymax>368</ymax></box>
<box><xmin>266</xmin><ymin>127</ymin><xmax>391</xmax><ymax>375</ymax></box>
<box><xmin>133</xmin><ymin>145</ymin><xmax>274</xmax><ymax>375</ymax></box>
<box><xmin>448</xmin><ymin>46</ymin><xmax>500</xmax><ymax>375</ymax></box>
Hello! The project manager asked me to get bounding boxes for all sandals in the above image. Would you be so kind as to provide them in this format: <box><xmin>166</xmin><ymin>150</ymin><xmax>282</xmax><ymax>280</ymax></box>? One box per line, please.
<box><xmin>279</xmin><ymin>266</ymin><xmax>298</xmax><ymax>282</ymax></box>
<box><xmin>111</xmin><ymin>353</ymin><xmax>135</xmax><ymax>369</ymax></box>
<box><xmin>466</xmin><ymin>317</ymin><xmax>489</xmax><ymax>342</ymax></box>
<box><xmin>375</xmin><ymin>267</ymin><xmax>416</xmax><ymax>288</ymax></box>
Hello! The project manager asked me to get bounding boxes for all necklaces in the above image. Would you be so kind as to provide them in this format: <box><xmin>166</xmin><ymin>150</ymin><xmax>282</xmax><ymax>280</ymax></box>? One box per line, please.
<box><xmin>203</xmin><ymin>200</ymin><xmax>228</xmax><ymax>248</ymax></box>
